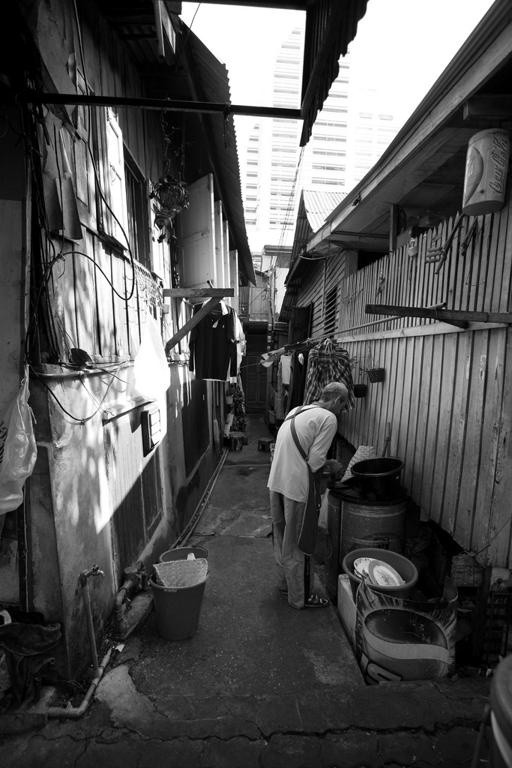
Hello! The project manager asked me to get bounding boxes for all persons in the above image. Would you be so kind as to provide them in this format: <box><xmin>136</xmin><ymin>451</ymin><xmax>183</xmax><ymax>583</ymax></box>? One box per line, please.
<box><xmin>268</xmin><ymin>383</ymin><xmax>348</xmax><ymax>608</ymax></box>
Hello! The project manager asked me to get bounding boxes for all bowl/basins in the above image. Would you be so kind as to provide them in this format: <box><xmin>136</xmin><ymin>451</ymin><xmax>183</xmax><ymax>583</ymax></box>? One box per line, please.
<box><xmin>160</xmin><ymin>546</ymin><xmax>209</xmax><ymax>563</ymax></box>
<box><xmin>351</xmin><ymin>457</ymin><xmax>404</xmax><ymax>491</ymax></box>
<box><xmin>342</xmin><ymin>547</ymin><xmax>419</xmax><ymax>593</ymax></box>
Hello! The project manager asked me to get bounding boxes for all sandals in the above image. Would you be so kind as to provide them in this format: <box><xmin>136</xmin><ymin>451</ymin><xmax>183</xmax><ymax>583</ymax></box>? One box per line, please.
<box><xmin>303</xmin><ymin>594</ymin><xmax>330</xmax><ymax>609</ymax></box>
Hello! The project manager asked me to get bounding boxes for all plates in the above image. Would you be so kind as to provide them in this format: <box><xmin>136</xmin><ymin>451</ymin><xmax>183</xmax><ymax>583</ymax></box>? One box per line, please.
<box><xmin>353</xmin><ymin>557</ymin><xmax>375</xmax><ymax>579</ymax></box>
<box><xmin>371</xmin><ymin>560</ymin><xmax>405</xmax><ymax>586</ymax></box>
<box><xmin>354</xmin><ymin>568</ymin><xmax>362</xmax><ymax>578</ymax></box>
